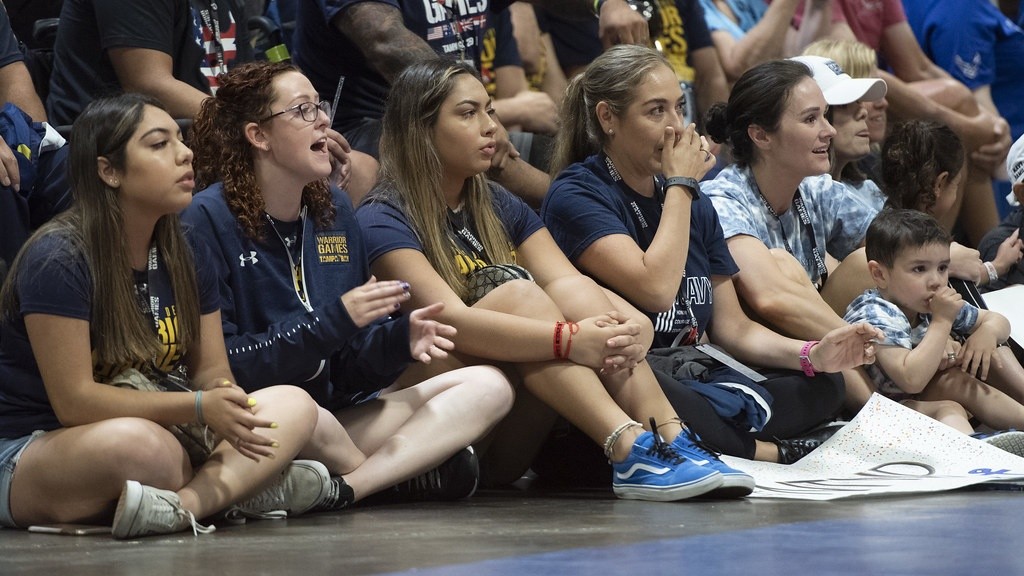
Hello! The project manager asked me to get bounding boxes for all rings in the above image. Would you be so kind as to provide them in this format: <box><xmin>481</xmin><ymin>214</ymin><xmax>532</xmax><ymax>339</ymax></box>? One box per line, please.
<box><xmin>234</xmin><ymin>437</ymin><xmax>240</xmax><ymax>446</ymax></box>
<box><xmin>947</xmin><ymin>353</ymin><xmax>956</xmax><ymax>360</ymax></box>
<box><xmin>700</xmin><ymin>148</ymin><xmax>712</xmax><ymax>162</ymax></box>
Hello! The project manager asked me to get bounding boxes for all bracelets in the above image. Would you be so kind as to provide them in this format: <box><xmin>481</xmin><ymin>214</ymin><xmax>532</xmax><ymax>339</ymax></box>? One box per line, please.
<box><xmin>799</xmin><ymin>340</ymin><xmax>821</xmax><ymax>376</ymax></box>
<box><xmin>664</xmin><ymin>176</ymin><xmax>701</xmax><ymax>201</ymax></box>
<box><xmin>984</xmin><ymin>261</ymin><xmax>999</xmax><ymax>286</ymax></box>
<box><xmin>194</xmin><ymin>390</ymin><xmax>207</xmax><ymax>428</ymax></box>
<box><xmin>554</xmin><ymin>321</ymin><xmax>579</xmax><ymax>362</ymax></box>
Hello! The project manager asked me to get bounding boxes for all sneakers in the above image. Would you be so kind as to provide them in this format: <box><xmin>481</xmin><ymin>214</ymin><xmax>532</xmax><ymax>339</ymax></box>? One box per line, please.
<box><xmin>234</xmin><ymin>460</ymin><xmax>354</xmax><ymax>520</ymax></box>
<box><xmin>670</xmin><ymin>417</ymin><xmax>755</xmax><ymax>498</ymax></box>
<box><xmin>771</xmin><ymin>420</ymin><xmax>851</xmax><ymax>464</ymax></box>
<box><xmin>968</xmin><ymin>427</ymin><xmax>1024</xmax><ymax>458</ymax></box>
<box><xmin>612</xmin><ymin>416</ymin><xmax>724</xmax><ymax>502</ymax></box>
<box><xmin>389</xmin><ymin>445</ymin><xmax>479</xmax><ymax>502</ymax></box>
<box><xmin>111</xmin><ymin>480</ymin><xmax>216</xmax><ymax>539</ymax></box>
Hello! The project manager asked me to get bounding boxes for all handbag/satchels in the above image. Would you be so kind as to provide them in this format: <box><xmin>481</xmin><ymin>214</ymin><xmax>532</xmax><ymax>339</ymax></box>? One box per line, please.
<box><xmin>108</xmin><ymin>367</ymin><xmax>214</xmax><ymax>466</ymax></box>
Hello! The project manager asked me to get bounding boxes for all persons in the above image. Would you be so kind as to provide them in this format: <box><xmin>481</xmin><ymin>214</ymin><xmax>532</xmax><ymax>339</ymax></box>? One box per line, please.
<box><xmin>176</xmin><ymin>60</ymin><xmax>513</xmax><ymax>519</ymax></box>
<box><xmin>0</xmin><ymin>0</ymin><xmax>1024</xmax><ymax>281</ymax></box>
<box><xmin>0</xmin><ymin>97</ymin><xmax>332</xmax><ymax>538</ymax></box>
<box><xmin>355</xmin><ymin>57</ymin><xmax>754</xmax><ymax>502</ymax></box>
<box><xmin>540</xmin><ymin>45</ymin><xmax>886</xmax><ymax>464</ymax></box>
<box><xmin>699</xmin><ymin>58</ymin><xmax>1024</xmax><ymax>456</ymax></box>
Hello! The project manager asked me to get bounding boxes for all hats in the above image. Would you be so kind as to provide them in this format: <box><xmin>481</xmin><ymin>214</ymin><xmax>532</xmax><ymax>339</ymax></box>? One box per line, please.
<box><xmin>784</xmin><ymin>56</ymin><xmax>887</xmax><ymax>105</ymax></box>
<box><xmin>1005</xmin><ymin>133</ymin><xmax>1024</xmax><ymax>207</ymax></box>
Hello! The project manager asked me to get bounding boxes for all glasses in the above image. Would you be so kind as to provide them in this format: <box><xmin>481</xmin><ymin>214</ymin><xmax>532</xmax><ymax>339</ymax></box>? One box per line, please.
<box><xmin>257</xmin><ymin>100</ymin><xmax>332</xmax><ymax>124</ymax></box>
